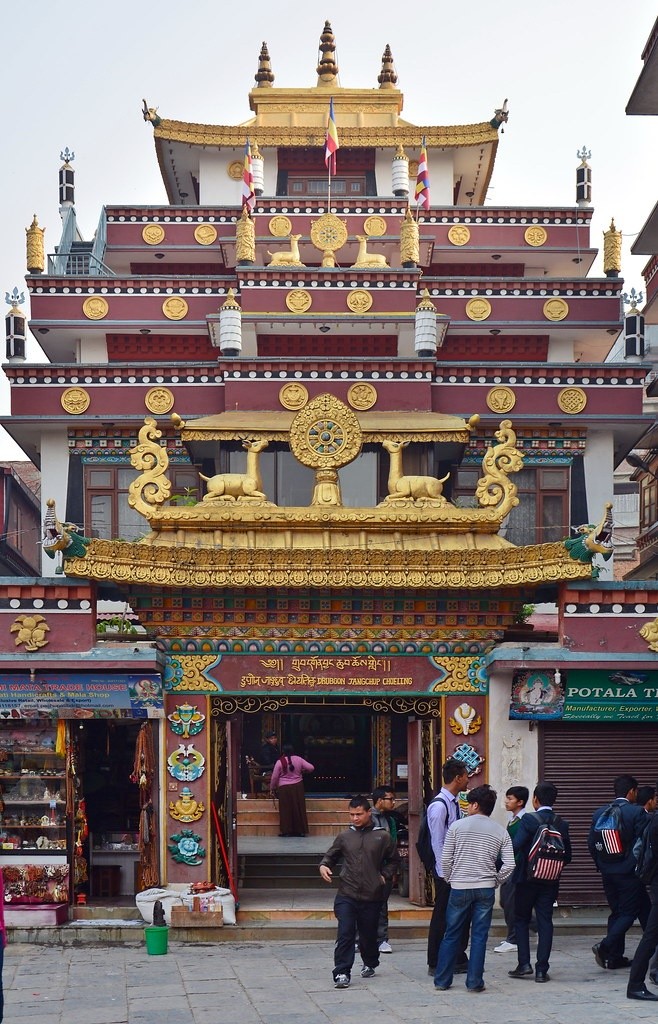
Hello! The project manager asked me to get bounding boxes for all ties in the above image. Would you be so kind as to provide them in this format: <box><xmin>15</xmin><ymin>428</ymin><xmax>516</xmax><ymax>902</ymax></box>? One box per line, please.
<box><xmin>453</xmin><ymin>799</ymin><xmax>460</xmax><ymax>820</ymax></box>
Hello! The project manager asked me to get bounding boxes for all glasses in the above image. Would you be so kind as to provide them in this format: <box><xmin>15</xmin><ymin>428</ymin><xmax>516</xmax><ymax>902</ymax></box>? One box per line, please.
<box><xmin>378</xmin><ymin>797</ymin><xmax>395</xmax><ymax>802</ymax></box>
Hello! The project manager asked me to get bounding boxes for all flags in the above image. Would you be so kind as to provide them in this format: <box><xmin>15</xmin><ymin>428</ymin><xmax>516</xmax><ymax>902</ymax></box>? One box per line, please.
<box><xmin>241</xmin><ymin>138</ymin><xmax>256</xmax><ymax>215</ymax></box>
<box><xmin>324</xmin><ymin>96</ymin><xmax>339</xmax><ymax>176</ymax></box>
<box><xmin>413</xmin><ymin>136</ymin><xmax>431</xmax><ymax>213</ymax></box>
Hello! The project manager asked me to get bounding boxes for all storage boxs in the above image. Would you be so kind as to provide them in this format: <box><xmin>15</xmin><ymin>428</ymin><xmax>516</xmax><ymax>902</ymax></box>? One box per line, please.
<box><xmin>171</xmin><ymin>905</ymin><xmax>224</xmax><ymax>928</ymax></box>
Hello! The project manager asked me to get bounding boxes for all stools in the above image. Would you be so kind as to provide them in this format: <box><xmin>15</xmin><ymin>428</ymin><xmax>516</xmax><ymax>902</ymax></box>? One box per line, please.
<box><xmin>91</xmin><ymin>864</ymin><xmax>122</xmax><ymax>896</ymax></box>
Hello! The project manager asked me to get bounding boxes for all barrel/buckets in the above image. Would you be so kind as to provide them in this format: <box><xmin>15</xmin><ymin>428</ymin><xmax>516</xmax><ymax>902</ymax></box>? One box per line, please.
<box><xmin>143</xmin><ymin>927</ymin><xmax>169</xmax><ymax>955</ymax></box>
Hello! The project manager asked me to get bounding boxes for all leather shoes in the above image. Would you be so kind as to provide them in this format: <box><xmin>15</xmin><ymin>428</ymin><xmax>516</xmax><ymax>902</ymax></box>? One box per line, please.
<box><xmin>535</xmin><ymin>972</ymin><xmax>550</xmax><ymax>982</ymax></box>
<box><xmin>592</xmin><ymin>942</ymin><xmax>606</xmax><ymax>969</ymax></box>
<box><xmin>608</xmin><ymin>957</ymin><xmax>633</xmax><ymax>969</ymax></box>
<box><xmin>508</xmin><ymin>963</ymin><xmax>533</xmax><ymax>976</ymax></box>
<box><xmin>627</xmin><ymin>989</ymin><xmax>658</xmax><ymax>1001</ymax></box>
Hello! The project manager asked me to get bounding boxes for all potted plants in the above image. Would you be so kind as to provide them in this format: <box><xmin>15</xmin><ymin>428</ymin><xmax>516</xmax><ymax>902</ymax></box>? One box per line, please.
<box><xmin>508</xmin><ymin>604</ymin><xmax>535</xmax><ymax>631</ymax></box>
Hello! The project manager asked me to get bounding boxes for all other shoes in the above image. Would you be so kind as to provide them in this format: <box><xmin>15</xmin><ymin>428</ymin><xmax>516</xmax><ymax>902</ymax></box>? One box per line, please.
<box><xmin>437</xmin><ymin>985</ymin><xmax>447</xmax><ymax>990</ymax></box>
<box><xmin>279</xmin><ymin>832</ymin><xmax>293</xmax><ymax>837</ymax></box>
<box><xmin>293</xmin><ymin>832</ymin><xmax>305</xmax><ymax>837</ymax></box>
<box><xmin>453</xmin><ymin>967</ymin><xmax>469</xmax><ymax>974</ymax></box>
<box><xmin>649</xmin><ymin>972</ymin><xmax>658</xmax><ymax>984</ymax></box>
<box><xmin>428</xmin><ymin>967</ymin><xmax>437</xmax><ymax>976</ymax></box>
<box><xmin>468</xmin><ymin>986</ymin><xmax>485</xmax><ymax>992</ymax></box>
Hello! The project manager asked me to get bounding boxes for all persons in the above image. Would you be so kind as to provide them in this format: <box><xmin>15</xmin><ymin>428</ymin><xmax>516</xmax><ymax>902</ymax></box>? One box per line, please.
<box><xmin>432</xmin><ymin>783</ymin><xmax>517</xmax><ymax>995</ymax></box>
<box><xmin>587</xmin><ymin>774</ymin><xmax>650</xmax><ymax>970</ymax></box>
<box><xmin>495</xmin><ymin>779</ymin><xmax>572</xmax><ymax>984</ymax></box>
<box><xmin>260</xmin><ymin>730</ymin><xmax>318</xmax><ymax>834</ymax></box>
<box><xmin>318</xmin><ymin>758</ymin><xmax>658</xmax><ymax>1002</ymax></box>
<box><xmin>318</xmin><ymin>795</ymin><xmax>402</xmax><ymax>989</ymax></box>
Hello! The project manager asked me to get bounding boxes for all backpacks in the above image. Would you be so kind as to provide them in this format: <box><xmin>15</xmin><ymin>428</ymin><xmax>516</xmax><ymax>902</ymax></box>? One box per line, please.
<box><xmin>592</xmin><ymin>803</ymin><xmax>639</xmax><ymax>863</ymax></box>
<box><xmin>633</xmin><ymin>811</ymin><xmax>658</xmax><ymax>884</ymax></box>
<box><xmin>415</xmin><ymin>797</ymin><xmax>448</xmax><ymax>871</ymax></box>
<box><xmin>525</xmin><ymin>812</ymin><xmax>566</xmax><ymax>886</ymax></box>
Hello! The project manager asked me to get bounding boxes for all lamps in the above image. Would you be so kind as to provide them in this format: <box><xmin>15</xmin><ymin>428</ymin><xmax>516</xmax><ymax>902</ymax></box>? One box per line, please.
<box><xmin>626</xmin><ymin>452</ymin><xmax>658</xmax><ymax>481</ymax></box>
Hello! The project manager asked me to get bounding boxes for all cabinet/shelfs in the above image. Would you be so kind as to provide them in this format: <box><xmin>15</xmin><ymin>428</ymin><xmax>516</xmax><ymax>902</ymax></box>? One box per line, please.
<box><xmin>0</xmin><ymin>726</ymin><xmax>70</xmax><ymax>902</ymax></box>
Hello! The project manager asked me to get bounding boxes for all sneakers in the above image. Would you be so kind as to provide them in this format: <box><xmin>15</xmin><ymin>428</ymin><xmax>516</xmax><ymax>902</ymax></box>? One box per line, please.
<box><xmin>494</xmin><ymin>941</ymin><xmax>518</xmax><ymax>952</ymax></box>
<box><xmin>354</xmin><ymin>944</ymin><xmax>360</xmax><ymax>953</ymax></box>
<box><xmin>360</xmin><ymin>965</ymin><xmax>375</xmax><ymax>977</ymax></box>
<box><xmin>379</xmin><ymin>940</ymin><xmax>392</xmax><ymax>953</ymax></box>
<box><xmin>334</xmin><ymin>973</ymin><xmax>350</xmax><ymax>988</ymax></box>
